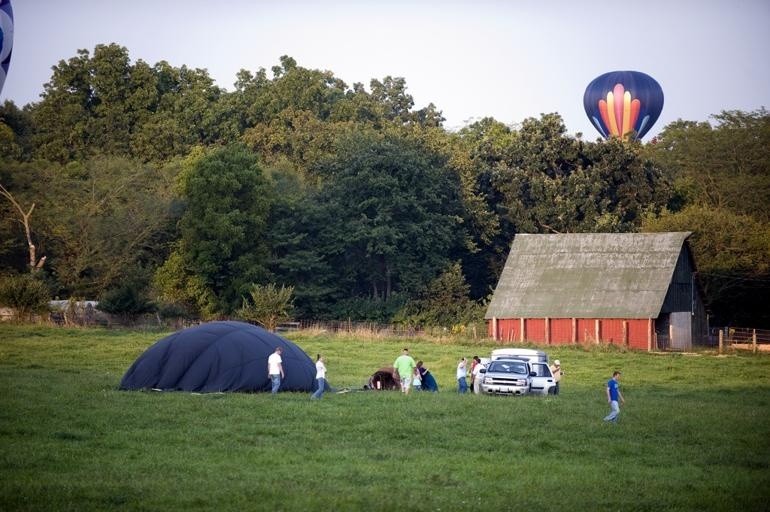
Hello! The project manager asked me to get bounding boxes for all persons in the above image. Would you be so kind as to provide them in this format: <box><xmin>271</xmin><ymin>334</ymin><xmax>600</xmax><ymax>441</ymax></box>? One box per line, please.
<box><xmin>267</xmin><ymin>345</ymin><xmax>285</xmax><ymax>394</ymax></box>
<box><xmin>456</xmin><ymin>356</ymin><xmax>486</xmax><ymax>395</ymax></box>
<box><xmin>551</xmin><ymin>359</ymin><xmax>564</xmax><ymax>395</ymax></box>
<box><xmin>311</xmin><ymin>353</ymin><xmax>327</xmax><ymax>400</ymax></box>
<box><xmin>603</xmin><ymin>371</ymin><xmax>625</xmax><ymax>422</ymax></box>
<box><xmin>392</xmin><ymin>348</ymin><xmax>428</xmax><ymax>395</ymax></box>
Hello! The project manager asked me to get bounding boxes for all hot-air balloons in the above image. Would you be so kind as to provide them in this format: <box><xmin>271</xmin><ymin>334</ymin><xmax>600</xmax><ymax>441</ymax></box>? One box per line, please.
<box><xmin>583</xmin><ymin>68</ymin><xmax>664</xmax><ymax>143</ymax></box>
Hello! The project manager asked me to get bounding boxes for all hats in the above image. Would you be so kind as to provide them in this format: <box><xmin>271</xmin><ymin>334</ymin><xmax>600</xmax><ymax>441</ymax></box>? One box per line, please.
<box><xmin>554</xmin><ymin>360</ymin><xmax>560</xmax><ymax>366</ymax></box>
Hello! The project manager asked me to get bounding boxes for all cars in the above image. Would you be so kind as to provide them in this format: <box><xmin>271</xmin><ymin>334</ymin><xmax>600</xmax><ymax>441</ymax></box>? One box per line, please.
<box><xmin>480</xmin><ymin>347</ymin><xmax>556</xmax><ymax>397</ymax></box>
